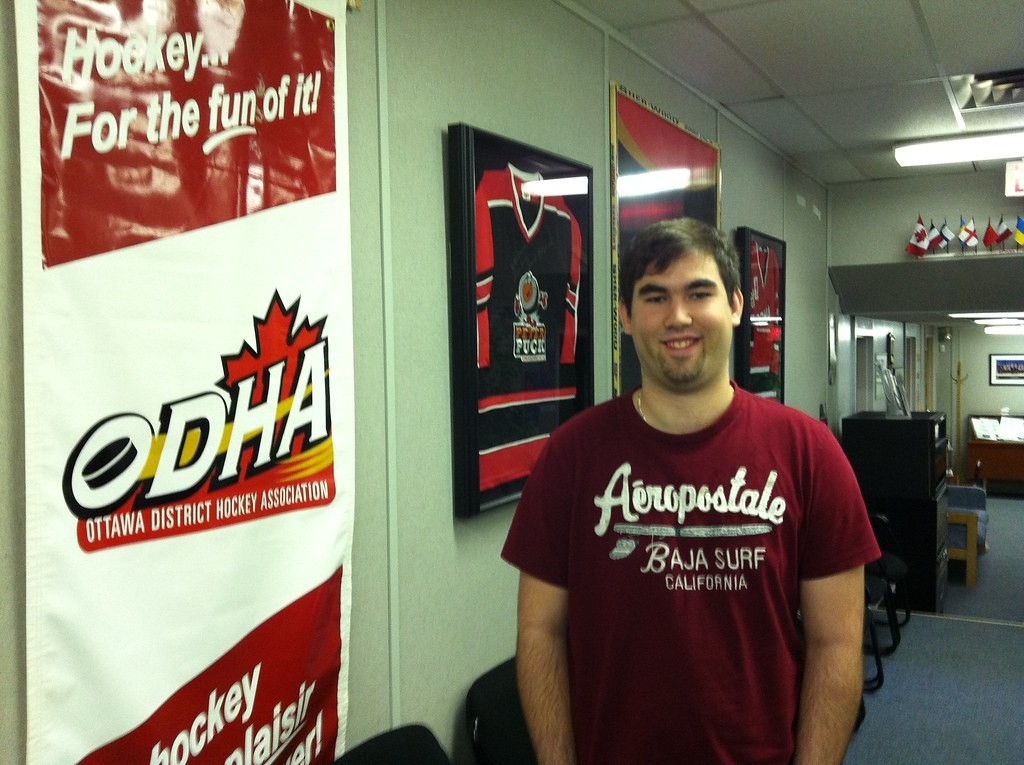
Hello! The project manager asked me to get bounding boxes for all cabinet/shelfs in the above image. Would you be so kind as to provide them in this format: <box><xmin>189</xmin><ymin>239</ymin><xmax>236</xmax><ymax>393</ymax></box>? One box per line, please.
<box><xmin>964</xmin><ymin>409</ymin><xmax>1024</xmax><ymax>496</ymax></box>
<box><xmin>840</xmin><ymin>408</ymin><xmax>951</xmax><ymax>616</ymax></box>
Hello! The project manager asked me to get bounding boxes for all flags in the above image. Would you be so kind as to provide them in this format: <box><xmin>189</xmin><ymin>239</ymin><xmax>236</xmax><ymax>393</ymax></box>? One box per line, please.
<box><xmin>1012</xmin><ymin>216</ymin><xmax>1024</xmax><ymax>245</ymax></box>
<box><xmin>905</xmin><ymin>216</ymin><xmax>930</xmax><ymax>257</ymax></box>
<box><xmin>995</xmin><ymin>221</ymin><xmax>1012</xmax><ymax>244</ymax></box>
<box><xmin>958</xmin><ymin>218</ymin><xmax>979</xmax><ymax>253</ymax></box>
<box><xmin>983</xmin><ymin>224</ymin><xmax>998</xmax><ymax>248</ymax></box>
<box><xmin>928</xmin><ymin>223</ymin><xmax>943</xmax><ymax>251</ymax></box>
<box><xmin>937</xmin><ymin>225</ymin><xmax>956</xmax><ymax>249</ymax></box>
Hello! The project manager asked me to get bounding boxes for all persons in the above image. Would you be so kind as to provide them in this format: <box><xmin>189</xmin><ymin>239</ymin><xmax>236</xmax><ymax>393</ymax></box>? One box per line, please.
<box><xmin>501</xmin><ymin>218</ymin><xmax>882</xmax><ymax>765</ymax></box>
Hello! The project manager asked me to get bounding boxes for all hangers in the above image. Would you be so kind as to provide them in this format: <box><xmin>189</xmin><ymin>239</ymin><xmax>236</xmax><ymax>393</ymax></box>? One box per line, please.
<box><xmin>948</xmin><ymin>361</ymin><xmax>971</xmax><ymax>486</ymax></box>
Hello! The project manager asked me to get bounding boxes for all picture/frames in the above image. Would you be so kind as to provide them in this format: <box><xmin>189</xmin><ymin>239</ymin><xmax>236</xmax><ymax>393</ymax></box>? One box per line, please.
<box><xmin>989</xmin><ymin>353</ymin><xmax>1024</xmax><ymax>386</ymax></box>
<box><xmin>736</xmin><ymin>221</ymin><xmax>787</xmax><ymax>409</ymax></box>
<box><xmin>609</xmin><ymin>77</ymin><xmax>723</xmax><ymax>400</ymax></box>
<box><xmin>448</xmin><ymin>120</ymin><xmax>596</xmax><ymax>518</ymax></box>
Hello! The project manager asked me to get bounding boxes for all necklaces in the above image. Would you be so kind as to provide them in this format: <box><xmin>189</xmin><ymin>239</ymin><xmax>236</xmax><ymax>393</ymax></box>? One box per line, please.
<box><xmin>638</xmin><ymin>394</ymin><xmax>647</xmax><ymax>423</ymax></box>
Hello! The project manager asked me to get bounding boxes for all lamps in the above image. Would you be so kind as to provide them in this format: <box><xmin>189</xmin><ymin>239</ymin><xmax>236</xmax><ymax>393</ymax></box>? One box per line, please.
<box><xmin>891</xmin><ymin>126</ymin><xmax>1024</xmax><ymax>168</ymax></box>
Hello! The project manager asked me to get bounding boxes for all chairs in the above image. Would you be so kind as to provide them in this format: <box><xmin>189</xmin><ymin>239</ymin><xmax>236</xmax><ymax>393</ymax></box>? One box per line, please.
<box><xmin>943</xmin><ymin>483</ymin><xmax>989</xmax><ymax>590</ymax></box>
